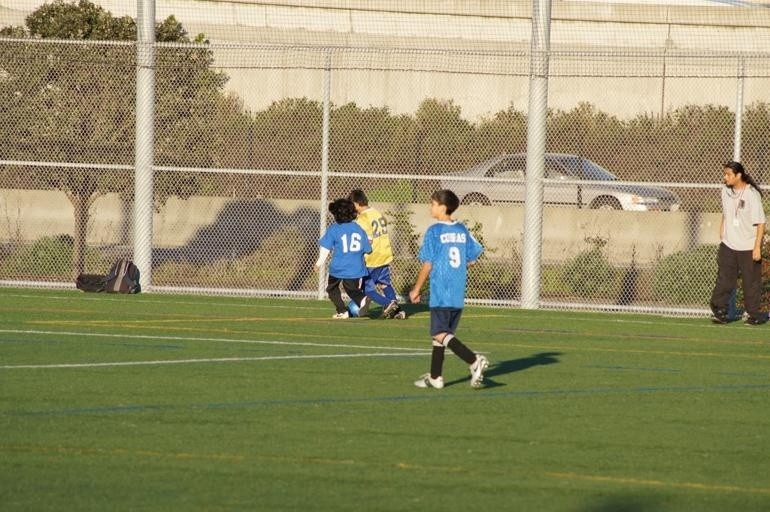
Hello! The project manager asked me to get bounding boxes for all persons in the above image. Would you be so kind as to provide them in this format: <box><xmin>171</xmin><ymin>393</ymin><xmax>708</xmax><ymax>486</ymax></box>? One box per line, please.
<box><xmin>709</xmin><ymin>161</ymin><xmax>766</xmax><ymax>327</ymax></box>
<box><xmin>410</xmin><ymin>188</ymin><xmax>491</xmax><ymax>389</ymax></box>
<box><xmin>348</xmin><ymin>190</ymin><xmax>406</xmax><ymax>319</ymax></box>
<box><xmin>312</xmin><ymin>199</ymin><xmax>372</xmax><ymax>321</ymax></box>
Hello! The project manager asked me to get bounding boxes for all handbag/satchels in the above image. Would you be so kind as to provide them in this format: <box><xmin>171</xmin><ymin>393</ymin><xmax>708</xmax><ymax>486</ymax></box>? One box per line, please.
<box><xmin>75</xmin><ymin>271</ymin><xmax>106</xmax><ymax>294</ymax></box>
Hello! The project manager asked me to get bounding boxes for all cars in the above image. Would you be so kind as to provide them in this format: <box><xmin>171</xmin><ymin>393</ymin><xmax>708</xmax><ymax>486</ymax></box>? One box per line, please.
<box><xmin>438</xmin><ymin>151</ymin><xmax>683</xmax><ymax>213</ymax></box>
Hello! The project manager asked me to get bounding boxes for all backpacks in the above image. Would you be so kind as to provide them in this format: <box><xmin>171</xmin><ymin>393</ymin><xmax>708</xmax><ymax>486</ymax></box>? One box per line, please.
<box><xmin>102</xmin><ymin>257</ymin><xmax>141</xmax><ymax>294</ymax></box>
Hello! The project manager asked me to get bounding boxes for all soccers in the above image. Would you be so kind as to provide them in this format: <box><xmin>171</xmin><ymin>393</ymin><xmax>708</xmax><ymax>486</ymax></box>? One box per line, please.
<box><xmin>347</xmin><ymin>300</ymin><xmax>360</xmax><ymax>317</ymax></box>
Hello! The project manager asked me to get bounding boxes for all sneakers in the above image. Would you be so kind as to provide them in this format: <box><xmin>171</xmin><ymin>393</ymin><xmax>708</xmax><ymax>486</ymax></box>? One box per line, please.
<box><xmin>332</xmin><ymin>310</ymin><xmax>349</xmax><ymax>319</ymax></box>
<box><xmin>743</xmin><ymin>316</ymin><xmax>768</xmax><ymax>326</ymax></box>
<box><xmin>711</xmin><ymin>308</ymin><xmax>729</xmax><ymax>324</ymax></box>
<box><xmin>381</xmin><ymin>299</ymin><xmax>406</xmax><ymax>319</ymax></box>
<box><xmin>468</xmin><ymin>353</ymin><xmax>490</xmax><ymax>388</ymax></box>
<box><xmin>413</xmin><ymin>372</ymin><xmax>444</xmax><ymax>389</ymax></box>
<box><xmin>358</xmin><ymin>294</ymin><xmax>372</xmax><ymax>317</ymax></box>
